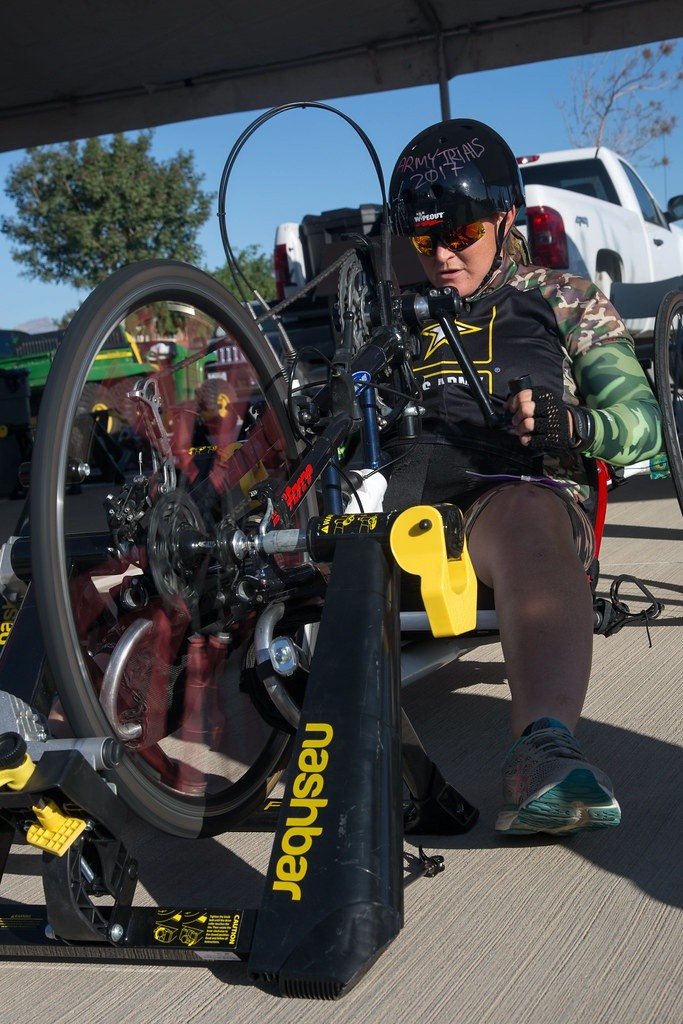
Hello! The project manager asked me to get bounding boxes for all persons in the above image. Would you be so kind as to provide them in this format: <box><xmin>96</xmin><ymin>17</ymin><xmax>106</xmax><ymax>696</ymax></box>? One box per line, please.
<box><xmin>382</xmin><ymin>120</ymin><xmax>662</xmax><ymax>837</ymax></box>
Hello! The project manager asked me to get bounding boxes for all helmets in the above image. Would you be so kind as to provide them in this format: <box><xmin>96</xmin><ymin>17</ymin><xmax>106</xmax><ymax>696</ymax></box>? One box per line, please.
<box><xmin>389</xmin><ymin>118</ymin><xmax>526</xmax><ymax>238</ymax></box>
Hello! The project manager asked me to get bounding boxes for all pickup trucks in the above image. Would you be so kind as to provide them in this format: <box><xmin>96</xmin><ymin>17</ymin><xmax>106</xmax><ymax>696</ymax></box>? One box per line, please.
<box><xmin>275</xmin><ymin>145</ymin><xmax>682</xmax><ymax>389</ymax></box>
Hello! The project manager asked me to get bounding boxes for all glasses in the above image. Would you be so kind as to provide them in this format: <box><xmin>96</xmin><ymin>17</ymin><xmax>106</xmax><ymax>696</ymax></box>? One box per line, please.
<box><xmin>408</xmin><ymin>219</ymin><xmax>485</xmax><ymax>257</ymax></box>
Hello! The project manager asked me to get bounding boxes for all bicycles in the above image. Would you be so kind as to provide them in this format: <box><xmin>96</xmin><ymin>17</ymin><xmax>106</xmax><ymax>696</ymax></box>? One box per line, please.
<box><xmin>25</xmin><ymin>104</ymin><xmax>533</xmax><ymax>843</ymax></box>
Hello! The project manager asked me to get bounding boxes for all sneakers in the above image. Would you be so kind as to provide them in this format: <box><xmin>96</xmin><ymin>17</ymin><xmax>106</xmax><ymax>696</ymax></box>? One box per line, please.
<box><xmin>495</xmin><ymin>716</ymin><xmax>621</xmax><ymax>834</ymax></box>
